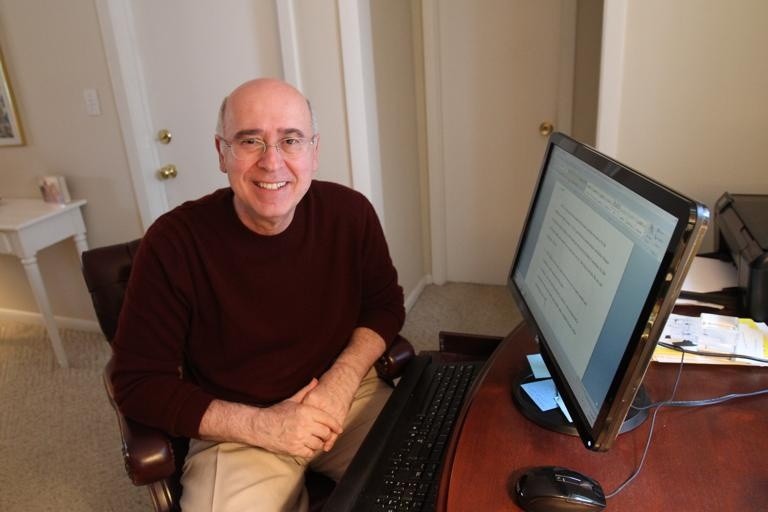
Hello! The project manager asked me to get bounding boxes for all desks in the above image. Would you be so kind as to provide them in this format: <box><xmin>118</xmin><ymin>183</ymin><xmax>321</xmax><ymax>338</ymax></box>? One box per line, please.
<box><xmin>0</xmin><ymin>199</ymin><xmax>89</xmax><ymax>378</ymax></box>
<box><xmin>436</xmin><ymin>251</ymin><xmax>768</xmax><ymax>511</ymax></box>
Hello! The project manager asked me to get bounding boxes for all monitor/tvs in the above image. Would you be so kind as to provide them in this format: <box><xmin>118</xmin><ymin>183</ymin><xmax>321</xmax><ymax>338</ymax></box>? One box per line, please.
<box><xmin>505</xmin><ymin>131</ymin><xmax>712</xmax><ymax>455</ymax></box>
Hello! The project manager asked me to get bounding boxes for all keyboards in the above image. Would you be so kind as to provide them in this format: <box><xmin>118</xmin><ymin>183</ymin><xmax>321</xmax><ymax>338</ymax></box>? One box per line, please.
<box><xmin>353</xmin><ymin>359</ymin><xmax>484</xmax><ymax>512</ymax></box>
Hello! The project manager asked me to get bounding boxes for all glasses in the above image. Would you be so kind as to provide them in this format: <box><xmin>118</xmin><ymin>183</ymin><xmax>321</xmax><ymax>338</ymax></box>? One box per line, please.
<box><xmin>220</xmin><ymin>135</ymin><xmax>314</xmax><ymax>162</ymax></box>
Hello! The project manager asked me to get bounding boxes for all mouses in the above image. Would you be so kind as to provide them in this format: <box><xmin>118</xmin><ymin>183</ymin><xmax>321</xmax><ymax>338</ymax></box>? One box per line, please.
<box><xmin>511</xmin><ymin>464</ymin><xmax>607</xmax><ymax>511</ymax></box>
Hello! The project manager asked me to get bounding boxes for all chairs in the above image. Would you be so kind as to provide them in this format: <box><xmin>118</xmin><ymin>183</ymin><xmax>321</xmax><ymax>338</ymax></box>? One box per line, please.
<box><xmin>81</xmin><ymin>238</ymin><xmax>414</xmax><ymax>512</ymax></box>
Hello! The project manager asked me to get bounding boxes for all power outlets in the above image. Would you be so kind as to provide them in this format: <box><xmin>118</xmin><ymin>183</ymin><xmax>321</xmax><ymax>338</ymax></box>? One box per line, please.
<box><xmin>84</xmin><ymin>89</ymin><xmax>101</xmax><ymax>117</ymax></box>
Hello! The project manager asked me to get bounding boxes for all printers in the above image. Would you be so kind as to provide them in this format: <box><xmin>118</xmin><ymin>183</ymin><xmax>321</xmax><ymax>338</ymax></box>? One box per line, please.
<box><xmin>680</xmin><ymin>190</ymin><xmax>768</xmax><ymax>294</ymax></box>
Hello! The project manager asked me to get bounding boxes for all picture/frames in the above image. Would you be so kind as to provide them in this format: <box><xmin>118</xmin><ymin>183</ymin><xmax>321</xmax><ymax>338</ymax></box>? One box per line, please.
<box><xmin>0</xmin><ymin>47</ymin><xmax>27</xmax><ymax>147</ymax></box>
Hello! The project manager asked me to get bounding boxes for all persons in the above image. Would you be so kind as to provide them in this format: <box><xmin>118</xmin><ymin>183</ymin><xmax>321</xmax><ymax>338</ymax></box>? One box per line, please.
<box><xmin>105</xmin><ymin>78</ymin><xmax>405</xmax><ymax>512</ymax></box>
